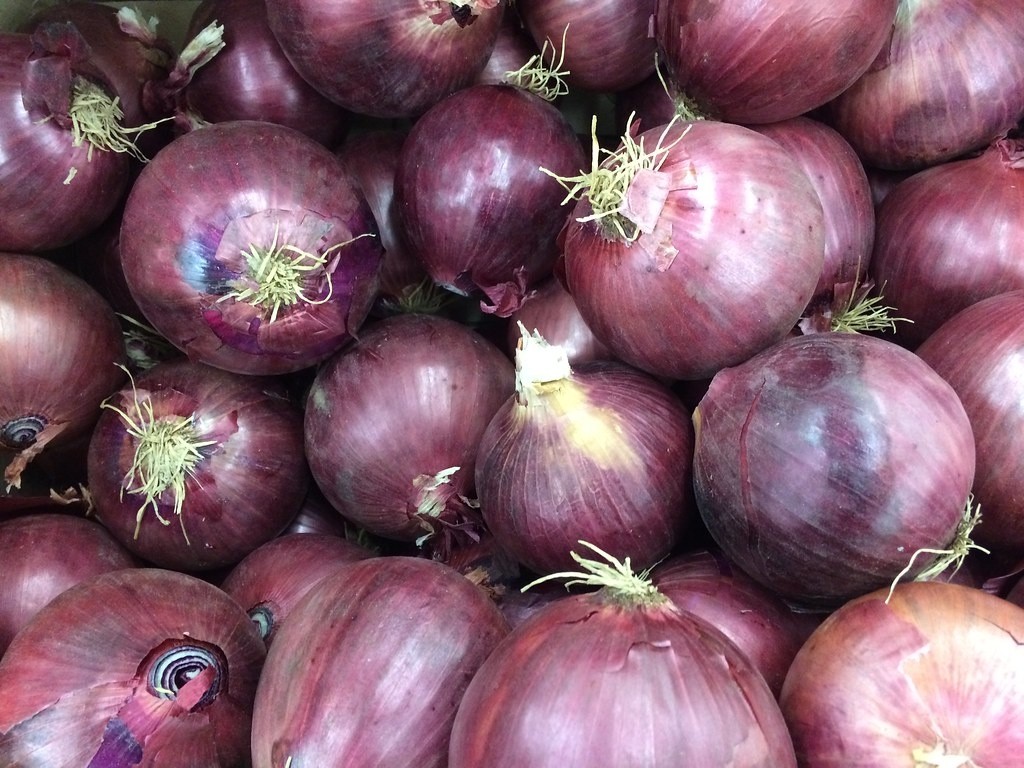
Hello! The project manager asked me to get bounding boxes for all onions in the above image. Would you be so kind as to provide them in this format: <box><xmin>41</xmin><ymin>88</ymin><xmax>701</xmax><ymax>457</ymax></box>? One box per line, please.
<box><xmin>0</xmin><ymin>0</ymin><xmax>1024</xmax><ymax>768</ymax></box>
